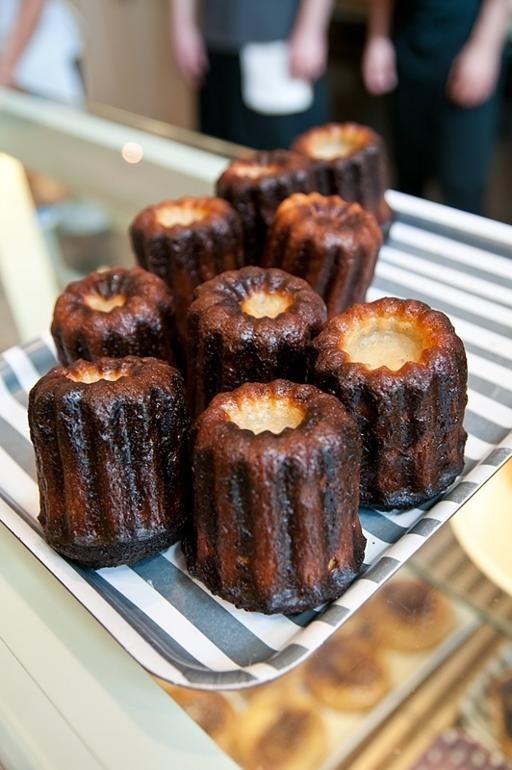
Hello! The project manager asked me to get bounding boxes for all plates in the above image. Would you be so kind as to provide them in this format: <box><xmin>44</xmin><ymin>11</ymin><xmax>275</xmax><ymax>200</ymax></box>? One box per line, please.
<box><xmin>0</xmin><ymin>185</ymin><xmax>512</xmax><ymax>693</ymax></box>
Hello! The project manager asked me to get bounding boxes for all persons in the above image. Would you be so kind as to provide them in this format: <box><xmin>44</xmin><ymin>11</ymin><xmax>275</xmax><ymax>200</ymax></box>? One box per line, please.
<box><xmin>167</xmin><ymin>0</ymin><xmax>335</xmax><ymax>153</ymax></box>
<box><xmin>359</xmin><ymin>0</ymin><xmax>511</xmax><ymax>216</ymax></box>
<box><xmin>0</xmin><ymin>0</ymin><xmax>88</xmax><ymax>113</ymax></box>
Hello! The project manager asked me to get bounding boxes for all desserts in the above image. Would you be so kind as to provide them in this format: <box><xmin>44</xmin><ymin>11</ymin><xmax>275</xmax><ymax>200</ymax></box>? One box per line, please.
<box><xmin>28</xmin><ymin>118</ymin><xmax>468</xmax><ymax>615</ymax></box>
